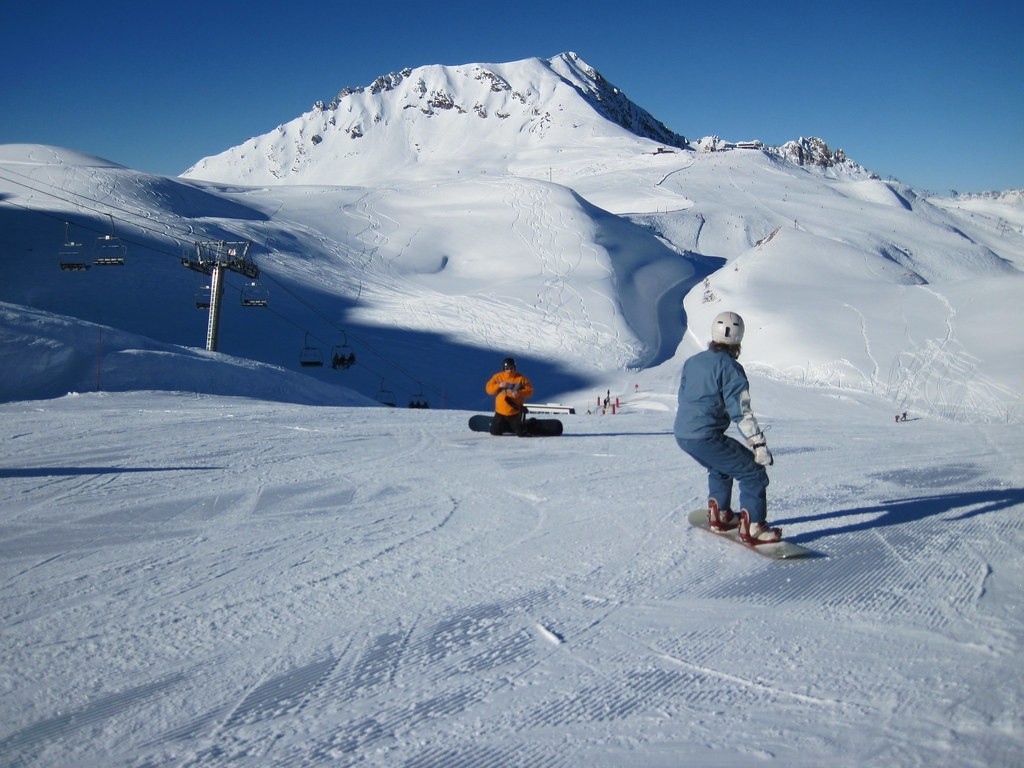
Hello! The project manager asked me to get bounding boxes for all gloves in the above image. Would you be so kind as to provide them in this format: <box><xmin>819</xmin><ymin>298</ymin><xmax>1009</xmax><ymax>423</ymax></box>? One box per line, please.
<box><xmin>746</xmin><ymin>425</ymin><xmax>774</xmax><ymax>466</ymax></box>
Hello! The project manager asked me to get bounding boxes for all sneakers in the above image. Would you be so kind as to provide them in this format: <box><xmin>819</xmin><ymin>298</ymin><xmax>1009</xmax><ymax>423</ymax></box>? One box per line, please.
<box><xmin>707</xmin><ymin>496</ymin><xmax>741</xmax><ymax>531</ymax></box>
<box><xmin>737</xmin><ymin>508</ymin><xmax>782</xmax><ymax>544</ymax></box>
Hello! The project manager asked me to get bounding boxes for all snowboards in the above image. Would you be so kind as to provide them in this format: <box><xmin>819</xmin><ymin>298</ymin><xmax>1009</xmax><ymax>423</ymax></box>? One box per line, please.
<box><xmin>688</xmin><ymin>508</ymin><xmax>816</xmax><ymax>561</ymax></box>
<box><xmin>470</xmin><ymin>416</ymin><xmax>563</xmax><ymax>438</ymax></box>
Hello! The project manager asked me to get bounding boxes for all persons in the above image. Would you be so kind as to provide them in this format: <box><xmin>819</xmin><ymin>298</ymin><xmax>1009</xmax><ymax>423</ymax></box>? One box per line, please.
<box><xmin>333</xmin><ymin>353</ymin><xmax>355</xmax><ymax>368</ymax></box>
<box><xmin>486</xmin><ymin>358</ymin><xmax>537</xmax><ymax>437</ymax></box>
<box><xmin>673</xmin><ymin>311</ymin><xmax>783</xmax><ymax>546</ymax></box>
<box><xmin>410</xmin><ymin>401</ymin><xmax>429</xmax><ymax>408</ymax></box>
<box><xmin>901</xmin><ymin>412</ymin><xmax>908</xmax><ymax>421</ymax></box>
<box><xmin>895</xmin><ymin>415</ymin><xmax>900</xmax><ymax>422</ymax></box>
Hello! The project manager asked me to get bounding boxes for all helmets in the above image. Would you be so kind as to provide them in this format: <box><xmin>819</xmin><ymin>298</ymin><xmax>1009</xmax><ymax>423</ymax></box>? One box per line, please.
<box><xmin>711</xmin><ymin>312</ymin><xmax>744</xmax><ymax>345</ymax></box>
<box><xmin>503</xmin><ymin>358</ymin><xmax>516</xmax><ymax>371</ymax></box>
